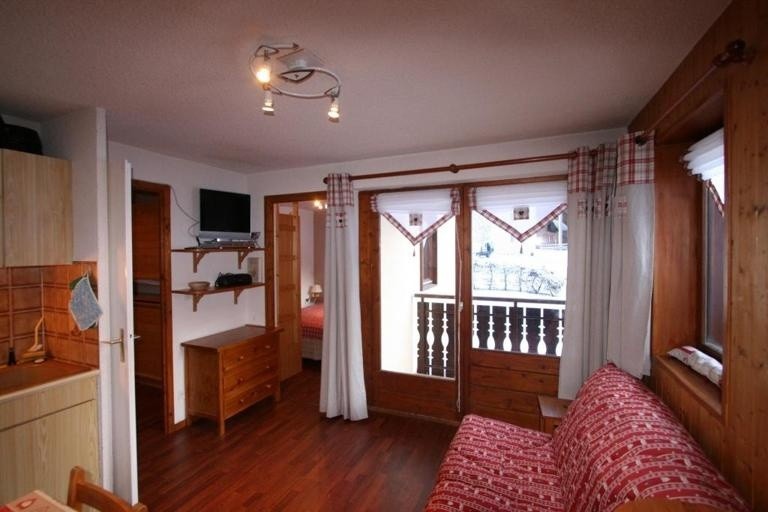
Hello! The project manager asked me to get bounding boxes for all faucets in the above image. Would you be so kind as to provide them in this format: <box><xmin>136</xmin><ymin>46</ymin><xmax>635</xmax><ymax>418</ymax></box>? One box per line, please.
<box><xmin>8</xmin><ymin>347</ymin><xmax>16</xmax><ymax>365</ymax></box>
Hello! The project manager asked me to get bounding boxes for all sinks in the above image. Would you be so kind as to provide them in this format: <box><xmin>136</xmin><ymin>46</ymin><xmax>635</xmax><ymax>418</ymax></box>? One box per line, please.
<box><xmin>0</xmin><ymin>359</ymin><xmax>92</xmax><ymax>395</ymax></box>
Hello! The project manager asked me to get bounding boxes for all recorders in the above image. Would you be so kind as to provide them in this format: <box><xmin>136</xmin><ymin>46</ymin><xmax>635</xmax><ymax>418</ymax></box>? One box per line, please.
<box><xmin>218</xmin><ymin>274</ymin><xmax>252</xmax><ymax>286</ymax></box>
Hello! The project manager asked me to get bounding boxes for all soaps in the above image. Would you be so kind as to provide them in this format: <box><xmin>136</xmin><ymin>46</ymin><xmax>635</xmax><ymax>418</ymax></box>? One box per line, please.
<box><xmin>34</xmin><ymin>358</ymin><xmax>44</xmax><ymax>363</ymax></box>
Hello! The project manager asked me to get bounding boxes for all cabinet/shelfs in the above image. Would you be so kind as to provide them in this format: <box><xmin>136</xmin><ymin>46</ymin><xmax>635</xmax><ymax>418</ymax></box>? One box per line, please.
<box><xmin>181</xmin><ymin>322</ymin><xmax>284</xmax><ymax>436</ymax></box>
<box><xmin>1</xmin><ymin>371</ymin><xmax>105</xmax><ymax>512</ymax></box>
<box><xmin>4</xmin><ymin>148</ymin><xmax>75</xmax><ymax>269</ymax></box>
<box><xmin>170</xmin><ymin>244</ymin><xmax>267</xmax><ymax>314</ymax></box>
<box><xmin>535</xmin><ymin>393</ymin><xmax>573</xmax><ymax>432</ymax></box>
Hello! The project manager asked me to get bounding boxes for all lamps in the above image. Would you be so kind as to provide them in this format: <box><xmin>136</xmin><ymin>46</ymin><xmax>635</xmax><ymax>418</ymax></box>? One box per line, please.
<box><xmin>311</xmin><ymin>283</ymin><xmax>322</xmax><ymax>298</ymax></box>
<box><xmin>249</xmin><ymin>41</ymin><xmax>342</xmax><ymax>121</ymax></box>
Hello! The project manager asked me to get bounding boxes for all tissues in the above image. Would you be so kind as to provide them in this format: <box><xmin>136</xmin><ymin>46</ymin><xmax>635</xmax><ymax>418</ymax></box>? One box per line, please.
<box><xmin>17</xmin><ymin>344</ymin><xmax>47</xmax><ymax>364</ymax></box>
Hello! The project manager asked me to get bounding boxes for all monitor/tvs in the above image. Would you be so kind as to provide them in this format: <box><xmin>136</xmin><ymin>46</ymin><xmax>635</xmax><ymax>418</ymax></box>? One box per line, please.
<box><xmin>199</xmin><ymin>189</ymin><xmax>250</xmax><ymax>243</ymax></box>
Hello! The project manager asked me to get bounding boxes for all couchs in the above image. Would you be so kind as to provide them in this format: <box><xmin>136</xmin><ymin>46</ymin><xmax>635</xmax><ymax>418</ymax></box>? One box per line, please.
<box><xmin>425</xmin><ymin>361</ymin><xmax>748</xmax><ymax>511</ymax></box>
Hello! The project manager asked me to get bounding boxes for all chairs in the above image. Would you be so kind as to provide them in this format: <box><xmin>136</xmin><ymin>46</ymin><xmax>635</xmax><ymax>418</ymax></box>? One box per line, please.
<box><xmin>65</xmin><ymin>462</ymin><xmax>146</xmax><ymax>512</ymax></box>
